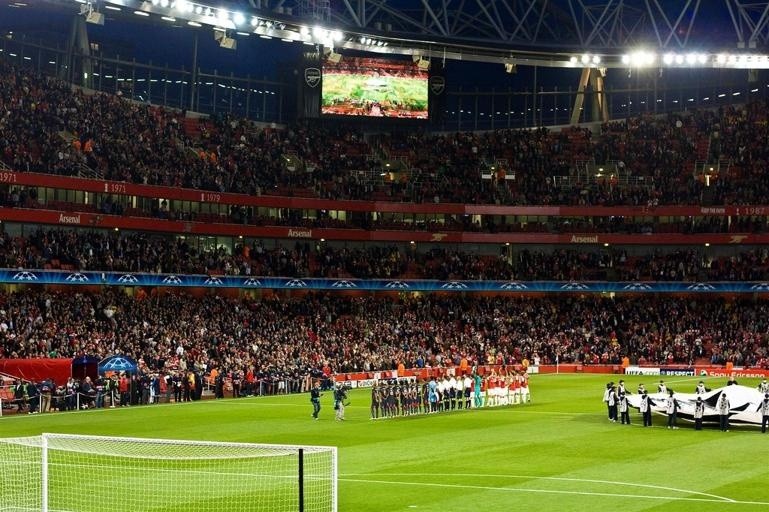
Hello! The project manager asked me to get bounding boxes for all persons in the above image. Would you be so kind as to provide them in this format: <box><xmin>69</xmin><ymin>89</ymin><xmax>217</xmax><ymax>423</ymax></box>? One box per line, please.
<box><xmin>694</xmin><ymin>397</ymin><xmax>705</xmax><ymax>430</ymax></box>
<box><xmin>603</xmin><ymin>384</ymin><xmax>630</xmax><ymax>424</ymax></box>
<box><xmin>3</xmin><ymin>65</ymin><xmax>769</xmax><ymax>414</ymax></box>
<box><xmin>666</xmin><ymin>391</ymin><xmax>681</xmax><ymax>430</ymax></box>
<box><xmin>718</xmin><ymin>393</ymin><xmax>730</xmax><ymax>433</ymax></box>
<box><xmin>310</xmin><ymin>382</ymin><xmax>323</xmax><ymax>420</ymax></box>
<box><xmin>369</xmin><ymin>380</ymin><xmax>421</xmax><ymax>420</ymax></box>
<box><xmin>333</xmin><ymin>381</ymin><xmax>349</xmax><ymax>422</ymax></box>
<box><xmin>641</xmin><ymin>391</ymin><xmax>656</xmax><ymax>427</ymax></box>
<box><xmin>755</xmin><ymin>394</ymin><xmax>769</xmax><ymax>433</ymax></box>
